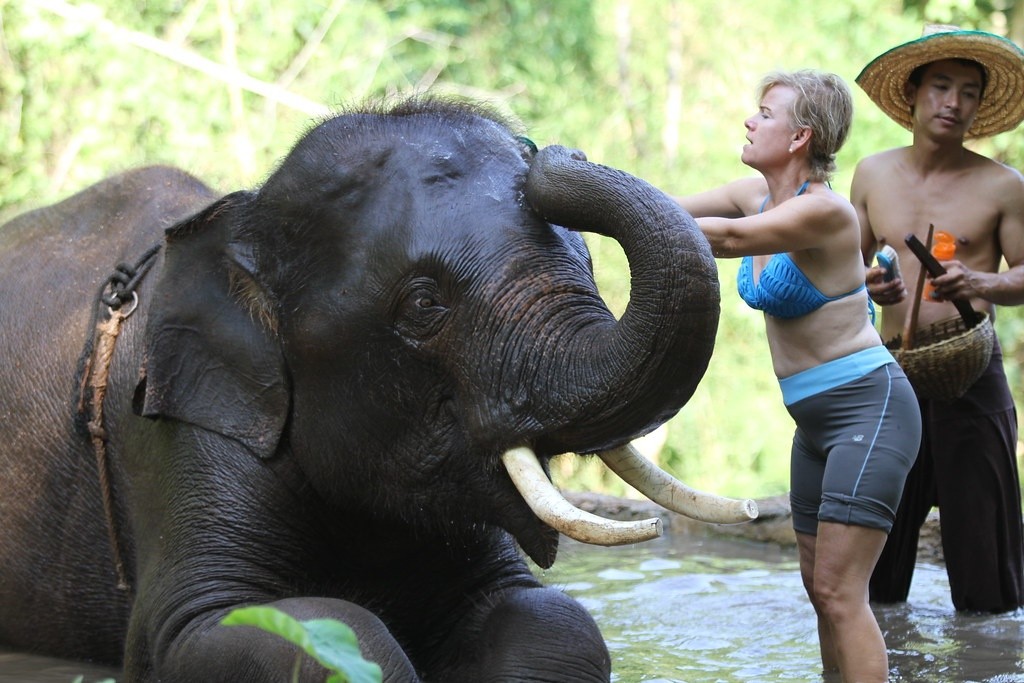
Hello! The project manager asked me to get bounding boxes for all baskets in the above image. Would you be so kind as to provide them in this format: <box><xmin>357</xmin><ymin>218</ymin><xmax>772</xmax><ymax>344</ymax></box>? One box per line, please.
<box><xmin>883</xmin><ymin>308</ymin><xmax>994</xmax><ymax>404</ymax></box>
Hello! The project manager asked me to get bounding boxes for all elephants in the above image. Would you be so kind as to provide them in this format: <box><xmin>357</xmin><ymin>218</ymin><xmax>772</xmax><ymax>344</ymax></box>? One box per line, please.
<box><xmin>0</xmin><ymin>90</ymin><xmax>722</xmax><ymax>683</ymax></box>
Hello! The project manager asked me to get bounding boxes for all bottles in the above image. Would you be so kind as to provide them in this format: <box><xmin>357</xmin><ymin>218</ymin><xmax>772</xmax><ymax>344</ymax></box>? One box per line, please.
<box><xmin>923</xmin><ymin>230</ymin><xmax>956</xmax><ymax>302</ymax></box>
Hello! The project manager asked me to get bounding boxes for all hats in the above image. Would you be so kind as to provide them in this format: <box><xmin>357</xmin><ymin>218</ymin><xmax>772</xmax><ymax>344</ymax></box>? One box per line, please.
<box><xmin>855</xmin><ymin>23</ymin><xmax>1024</xmax><ymax>142</ymax></box>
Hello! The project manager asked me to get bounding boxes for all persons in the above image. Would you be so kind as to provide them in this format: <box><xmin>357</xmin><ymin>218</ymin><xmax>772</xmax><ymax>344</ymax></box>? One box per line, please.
<box><xmin>847</xmin><ymin>25</ymin><xmax>1024</xmax><ymax>617</ymax></box>
<box><xmin>668</xmin><ymin>69</ymin><xmax>921</xmax><ymax>683</ymax></box>
